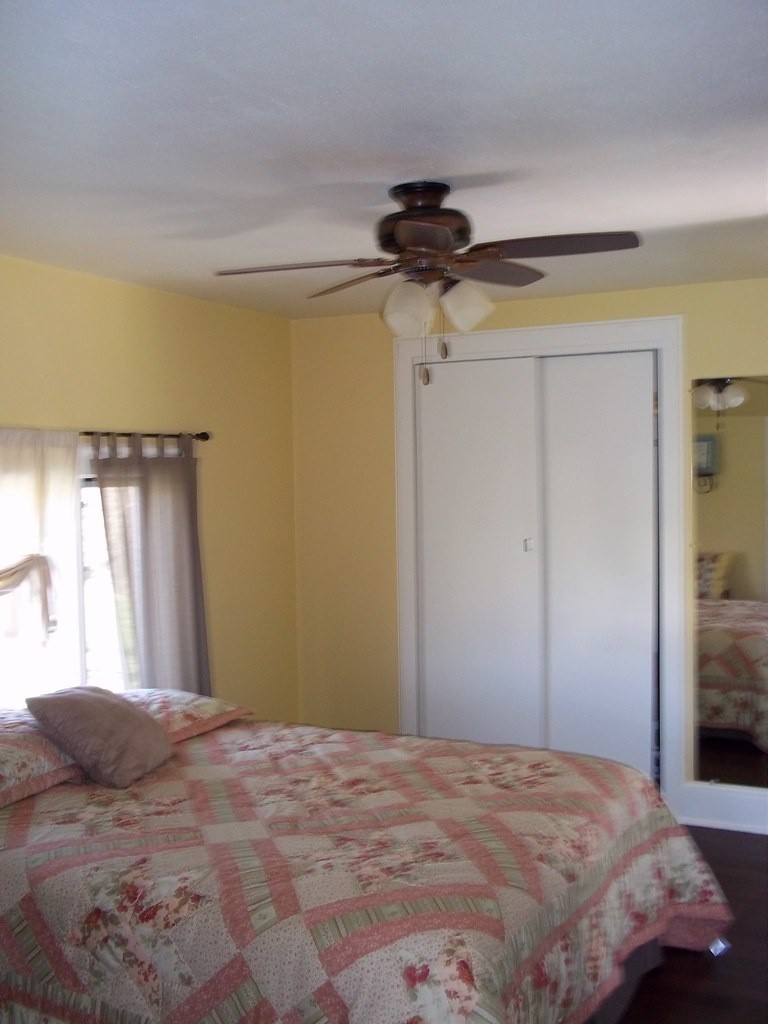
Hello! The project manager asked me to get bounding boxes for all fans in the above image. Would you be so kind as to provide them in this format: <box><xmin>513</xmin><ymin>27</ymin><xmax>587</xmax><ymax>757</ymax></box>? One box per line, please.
<box><xmin>214</xmin><ymin>181</ymin><xmax>639</xmax><ymax>300</ymax></box>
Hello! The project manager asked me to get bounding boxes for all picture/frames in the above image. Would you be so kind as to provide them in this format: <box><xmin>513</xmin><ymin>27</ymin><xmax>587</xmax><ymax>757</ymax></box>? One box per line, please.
<box><xmin>692</xmin><ymin>435</ymin><xmax>716</xmax><ymax>477</ymax></box>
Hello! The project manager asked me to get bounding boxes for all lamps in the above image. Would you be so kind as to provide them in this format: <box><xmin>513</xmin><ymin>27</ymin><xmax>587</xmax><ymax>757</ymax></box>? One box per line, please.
<box><xmin>694</xmin><ymin>383</ymin><xmax>744</xmax><ymax>429</ymax></box>
<box><xmin>383</xmin><ymin>271</ymin><xmax>495</xmax><ymax>381</ymax></box>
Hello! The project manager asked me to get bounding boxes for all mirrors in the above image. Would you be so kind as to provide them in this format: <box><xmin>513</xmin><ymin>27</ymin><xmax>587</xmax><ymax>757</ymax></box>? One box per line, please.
<box><xmin>687</xmin><ymin>374</ymin><xmax>767</xmax><ymax>788</ymax></box>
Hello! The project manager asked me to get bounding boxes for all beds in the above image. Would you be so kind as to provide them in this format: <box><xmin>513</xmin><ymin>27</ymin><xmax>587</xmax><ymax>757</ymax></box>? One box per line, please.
<box><xmin>692</xmin><ymin>600</ymin><xmax>766</xmax><ymax>750</ymax></box>
<box><xmin>0</xmin><ymin>684</ymin><xmax>734</xmax><ymax>1023</ymax></box>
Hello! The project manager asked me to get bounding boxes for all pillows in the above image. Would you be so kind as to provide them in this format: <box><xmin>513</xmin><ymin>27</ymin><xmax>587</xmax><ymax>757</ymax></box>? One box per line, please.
<box><xmin>1</xmin><ymin>709</ymin><xmax>84</xmax><ymax>809</ymax></box>
<box><xmin>26</xmin><ymin>685</ymin><xmax>176</xmax><ymax>789</ymax></box>
<box><xmin>696</xmin><ymin>549</ymin><xmax>737</xmax><ymax>599</ymax></box>
<box><xmin>115</xmin><ymin>690</ymin><xmax>252</xmax><ymax>744</ymax></box>
